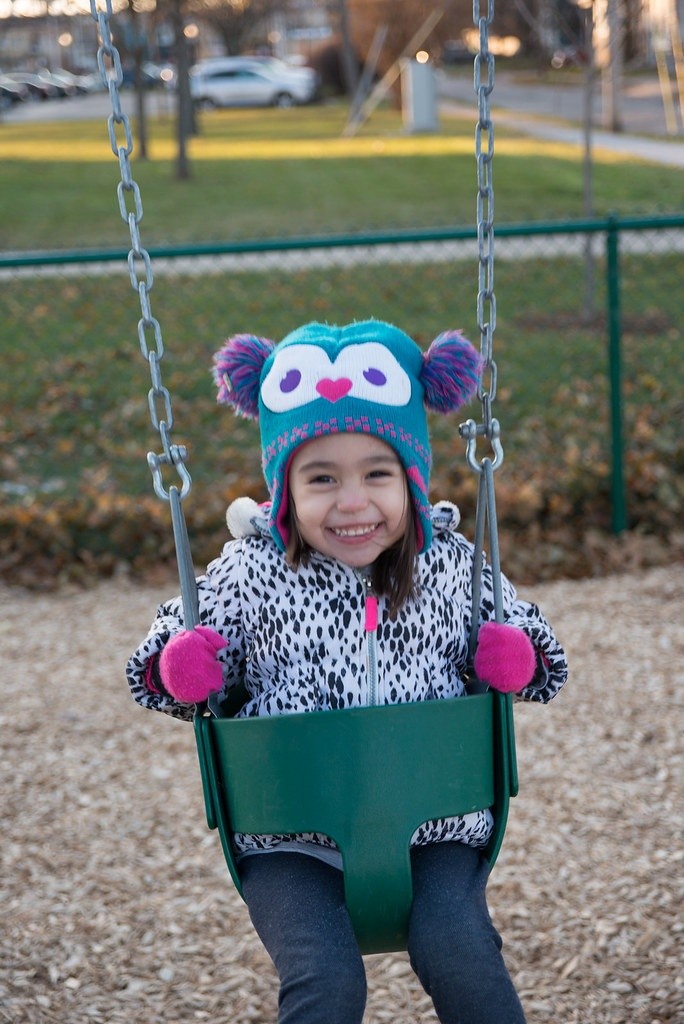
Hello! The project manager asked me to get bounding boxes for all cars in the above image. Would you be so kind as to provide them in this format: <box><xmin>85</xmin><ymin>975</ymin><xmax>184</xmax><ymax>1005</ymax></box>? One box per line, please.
<box><xmin>186</xmin><ymin>53</ymin><xmax>321</xmax><ymax>112</ymax></box>
<box><xmin>1</xmin><ymin>60</ymin><xmax>176</xmax><ymax>111</ymax></box>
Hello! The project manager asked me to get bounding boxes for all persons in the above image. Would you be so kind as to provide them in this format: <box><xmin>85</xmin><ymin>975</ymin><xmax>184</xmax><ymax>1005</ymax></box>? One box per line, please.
<box><xmin>124</xmin><ymin>319</ymin><xmax>570</xmax><ymax>1024</ymax></box>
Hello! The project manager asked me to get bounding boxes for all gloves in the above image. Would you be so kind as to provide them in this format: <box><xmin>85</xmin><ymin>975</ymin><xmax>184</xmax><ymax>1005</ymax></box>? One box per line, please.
<box><xmin>158</xmin><ymin>626</ymin><xmax>227</xmax><ymax>703</ymax></box>
<box><xmin>475</xmin><ymin>622</ymin><xmax>534</xmax><ymax>694</ymax></box>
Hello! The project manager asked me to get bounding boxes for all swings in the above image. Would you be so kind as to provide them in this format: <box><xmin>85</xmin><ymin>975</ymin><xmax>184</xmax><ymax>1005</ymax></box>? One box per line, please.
<box><xmin>86</xmin><ymin>0</ymin><xmax>524</xmax><ymax>958</ymax></box>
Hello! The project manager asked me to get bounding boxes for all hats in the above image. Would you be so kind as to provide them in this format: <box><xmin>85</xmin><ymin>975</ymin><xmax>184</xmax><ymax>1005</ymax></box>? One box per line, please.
<box><xmin>211</xmin><ymin>319</ymin><xmax>480</xmax><ymax>555</ymax></box>
